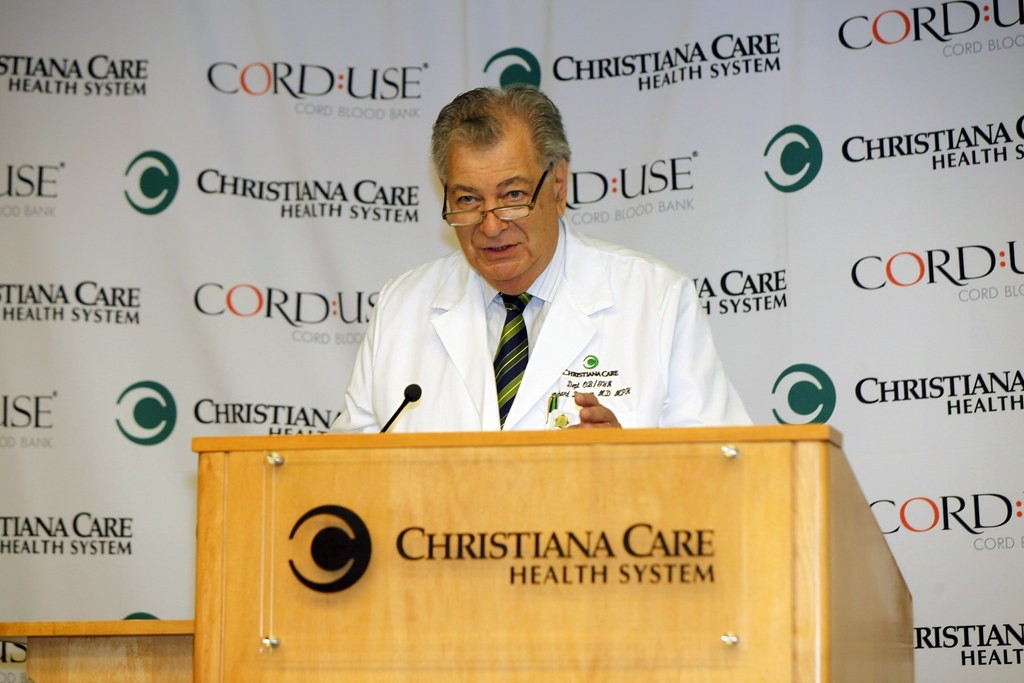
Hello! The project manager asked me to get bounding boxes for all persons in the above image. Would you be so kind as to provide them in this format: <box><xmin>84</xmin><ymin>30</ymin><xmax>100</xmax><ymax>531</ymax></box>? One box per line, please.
<box><xmin>328</xmin><ymin>86</ymin><xmax>754</xmax><ymax>433</ymax></box>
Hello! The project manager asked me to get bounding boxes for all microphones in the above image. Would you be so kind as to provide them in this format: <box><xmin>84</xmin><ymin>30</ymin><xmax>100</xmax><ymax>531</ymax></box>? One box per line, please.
<box><xmin>381</xmin><ymin>383</ymin><xmax>422</xmax><ymax>435</ymax></box>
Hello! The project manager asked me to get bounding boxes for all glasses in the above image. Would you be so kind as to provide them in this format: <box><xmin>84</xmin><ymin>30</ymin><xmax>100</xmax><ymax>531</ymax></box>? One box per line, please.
<box><xmin>442</xmin><ymin>164</ymin><xmax>552</xmax><ymax>226</ymax></box>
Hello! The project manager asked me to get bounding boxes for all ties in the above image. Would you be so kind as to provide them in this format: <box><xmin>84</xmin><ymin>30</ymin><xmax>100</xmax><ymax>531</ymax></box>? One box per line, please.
<box><xmin>493</xmin><ymin>292</ymin><xmax>535</xmax><ymax>430</ymax></box>
